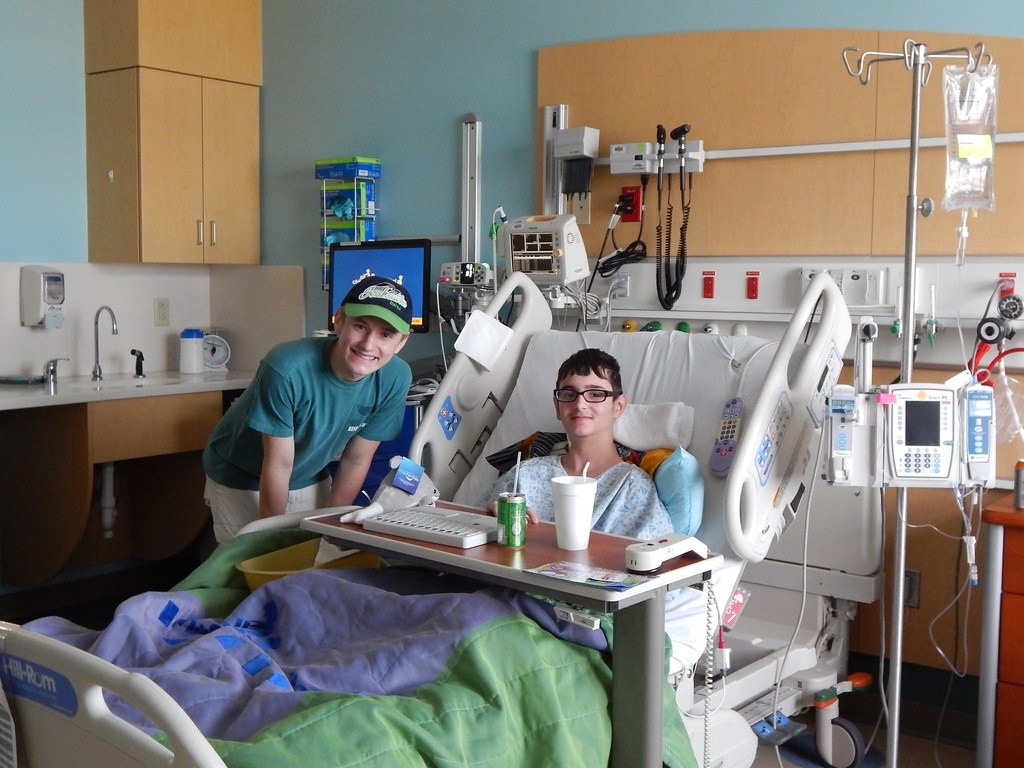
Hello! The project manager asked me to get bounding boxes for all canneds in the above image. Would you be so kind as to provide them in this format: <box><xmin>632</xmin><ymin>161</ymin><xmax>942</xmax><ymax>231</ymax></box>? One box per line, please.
<box><xmin>1014</xmin><ymin>459</ymin><xmax>1024</xmax><ymax>508</ymax></box>
<box><xmin>496</xmin><ymin>492</ymin><xmax>526</xmax><ymax>550</ymax></box>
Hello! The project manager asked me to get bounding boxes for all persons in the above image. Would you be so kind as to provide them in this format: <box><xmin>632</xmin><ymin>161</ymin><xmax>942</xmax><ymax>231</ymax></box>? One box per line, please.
<box><xmin>483</xmin><ymin>349</ymin><xmax>675</xmax><ymax>541</ymax></box>
<box><xmin>204</xmin><ymin>276</ymin><xmax>413</xmax><ymax>545</ymax></box>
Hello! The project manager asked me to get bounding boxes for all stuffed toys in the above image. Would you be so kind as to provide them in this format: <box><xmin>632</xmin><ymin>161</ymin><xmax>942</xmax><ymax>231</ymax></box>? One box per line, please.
<box><xmin>339</xmin><ymin>456</ymin><xmax>440</xmax><ymax>524</ymax></box>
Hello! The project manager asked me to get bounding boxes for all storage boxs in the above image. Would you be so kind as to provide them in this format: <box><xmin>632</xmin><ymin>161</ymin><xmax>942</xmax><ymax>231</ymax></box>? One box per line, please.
<box><xmin>315</xmin><ymin>157</ymin><xmax>381</xmax><ymax>290</ymax></box>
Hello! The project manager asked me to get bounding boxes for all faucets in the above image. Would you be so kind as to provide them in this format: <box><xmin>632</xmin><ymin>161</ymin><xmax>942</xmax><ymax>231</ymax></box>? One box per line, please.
<box><xmin>91</xmin><ymin>305</ymin><xmax>119</xmax><ymax>381</ymax></box>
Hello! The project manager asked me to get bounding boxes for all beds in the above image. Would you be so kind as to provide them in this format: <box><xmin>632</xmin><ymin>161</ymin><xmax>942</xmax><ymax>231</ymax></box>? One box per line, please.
<box><xmin>0</xmin><ymin>270</ymin><xmax>886</xmax><ymax>768</ymax></box>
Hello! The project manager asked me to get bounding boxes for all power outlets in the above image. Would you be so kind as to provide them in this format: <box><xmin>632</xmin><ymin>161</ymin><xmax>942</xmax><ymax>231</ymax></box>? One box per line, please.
<box><xmin>612</xmin><ymin>276</ymin><xmax>629</xmax><ymax>298</ymax></box>
<box><xmin>622</xmin><ymin>186</ymin><xmax>643</xmax><ymax>222</ymax></box>
<box><xmin>155</xmin><ymin>299</ymin><xmax>169</xmax><ymax>328</ymax></box>
<box><xmin>904</xmin><ymin>569</ymin><xmax>920</xmax><ymax>608</ymax></box>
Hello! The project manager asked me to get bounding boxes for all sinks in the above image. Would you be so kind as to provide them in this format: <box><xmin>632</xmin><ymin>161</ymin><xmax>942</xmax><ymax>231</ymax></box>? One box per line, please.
<box><xmin>69</xmin><ymin>379</ymin><xmax>188</xmax><ymax>389</ymax></box>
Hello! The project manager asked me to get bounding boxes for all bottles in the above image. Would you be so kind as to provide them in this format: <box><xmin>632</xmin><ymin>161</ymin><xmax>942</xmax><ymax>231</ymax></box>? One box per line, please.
<box><xmin>180</xmin><ymin>329</ymin><xmax>204</xmax><ymax>374</ymax></box>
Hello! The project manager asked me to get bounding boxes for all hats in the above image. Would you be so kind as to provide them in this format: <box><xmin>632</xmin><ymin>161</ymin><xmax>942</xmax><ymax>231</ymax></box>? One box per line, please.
<box><xmin>341</xmin><ymin>276</ymin><xmax>413</xmax><ymax>336</ymax></box>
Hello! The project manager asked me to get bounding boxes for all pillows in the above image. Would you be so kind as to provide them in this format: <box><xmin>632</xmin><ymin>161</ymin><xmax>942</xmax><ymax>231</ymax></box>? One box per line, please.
<box><xmin>653</xmin><ymin>444</ymin><xmax>705</xmax><ymax>537</ymax></box>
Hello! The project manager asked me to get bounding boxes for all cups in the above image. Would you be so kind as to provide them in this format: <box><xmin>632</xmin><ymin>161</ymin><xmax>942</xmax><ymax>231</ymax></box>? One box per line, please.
<box><xmin>550</xmin><ymin>476</ymin><xmax>598</xmax><ymax>551</ymax></box>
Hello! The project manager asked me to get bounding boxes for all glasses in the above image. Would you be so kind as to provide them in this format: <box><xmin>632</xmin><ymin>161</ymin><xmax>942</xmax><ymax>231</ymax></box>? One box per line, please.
<box><xmin>553</xmin><ymin>388</ymin><xmax>616</xmax><ymax>403</ymax></box>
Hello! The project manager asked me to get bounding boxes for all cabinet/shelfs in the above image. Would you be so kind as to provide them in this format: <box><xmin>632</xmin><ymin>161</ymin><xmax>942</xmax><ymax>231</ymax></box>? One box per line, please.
<box><xmin>321</xmin><ymin>178</ymin><xmax>381</xmax><ymax>292</ymax></box>
<box><xmin>86</xmin><ymin>67</ymin><xmax>259</xmax><ymax>266</ymax></box>
<box><xmin>976</xmin><ymin>492</ymin><xmax>1024</xmax><ymax>768</ymax></box>
<box><xmin>84</xmin><ymin>0</ymin><xmax>263</xmax><ymax>87</ymax></box>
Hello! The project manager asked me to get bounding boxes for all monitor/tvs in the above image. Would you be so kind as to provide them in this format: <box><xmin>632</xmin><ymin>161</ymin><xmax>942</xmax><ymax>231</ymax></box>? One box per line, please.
<box><xmin>329</xmin><ymin>239</ymin><xmax>431</xmax><ymax>333</ymax></box>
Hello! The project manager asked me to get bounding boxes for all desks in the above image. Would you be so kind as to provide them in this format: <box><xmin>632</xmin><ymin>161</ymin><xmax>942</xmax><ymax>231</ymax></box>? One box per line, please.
<box><xmin>301</xmin><ymin>499</ymin><xmax>724</xmax><ymax>768</ymax></box>
